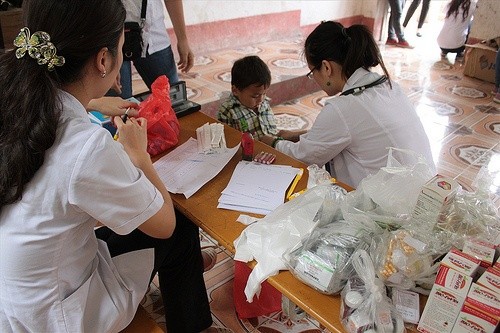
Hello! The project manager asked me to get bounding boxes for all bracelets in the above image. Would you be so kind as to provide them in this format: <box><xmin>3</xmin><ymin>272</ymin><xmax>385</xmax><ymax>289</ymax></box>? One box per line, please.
<box><xmin>270</xmin><ymin>136</ymin><xmax>285</xmax><ymax>147</ymax></box>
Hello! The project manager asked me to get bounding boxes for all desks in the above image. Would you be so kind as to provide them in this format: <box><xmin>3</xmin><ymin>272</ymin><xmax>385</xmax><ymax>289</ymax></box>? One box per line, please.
<box><xmin>134</xmin><ymin>112</ymin><xmax>357</xmax><ymax>333</ymax></box>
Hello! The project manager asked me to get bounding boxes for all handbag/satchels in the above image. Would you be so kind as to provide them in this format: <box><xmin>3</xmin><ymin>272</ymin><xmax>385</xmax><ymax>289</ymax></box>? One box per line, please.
<box><xmin>124</xmin><ymin>20</ymin><xmax>144</xmax><ymax>60</ymax></box>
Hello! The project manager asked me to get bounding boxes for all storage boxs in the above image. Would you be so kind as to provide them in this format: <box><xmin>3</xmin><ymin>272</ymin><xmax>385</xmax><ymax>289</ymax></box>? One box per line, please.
<box><xmin>462</xmin><ymin>34</ymin><xmax>500</xmax><ymax>84</ymax></box>
<box><xmin>411</xmin><ymin>174</ymin><xmax>500</xmax><ymax>333</ymax></box>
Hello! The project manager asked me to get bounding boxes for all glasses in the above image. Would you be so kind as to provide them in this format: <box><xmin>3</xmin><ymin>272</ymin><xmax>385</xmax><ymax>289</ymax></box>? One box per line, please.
<box><xmin>306</xmin><ymin>66</ymin><xmax>317</xmax><ymax>80</ymax></box>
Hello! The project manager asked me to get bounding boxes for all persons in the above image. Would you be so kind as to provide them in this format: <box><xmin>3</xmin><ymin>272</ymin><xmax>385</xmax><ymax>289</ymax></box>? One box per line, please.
<box><xmin>0</xmin><ymin>1</ymin><xmax>234</xmax><ymax>333</ymax></box>
<box><xmin>215</xmin><ymin>54</ymin><xmax>280</xmax><ymax>140</ymax></box>
<box><xmin>437</xmin><ymin>1</ymin><xmax>478</xmax><ymax>58</ymax></box>
<box><xmin>261</xmin><ymin>21</ymin><xmax>438</xmax><ymax>187</ymax></box>
<box><xmin>491</xmin><ymin>50</ymin><xmax>500</xmax><ymax>103</ymax></box>
<box><xmin>402</xmin><ymin>0</ymin><xmax>431</xmax><ymax>38</ymax></box>
<box><xmin>109</xmin><ymin>0</ymin><xmax>195</xmax><ymax>98</ymax></box>
<box><xmin>385</xmin><ymin>0</ymin><xmax>414</xmax><ymax>50</ymax></box>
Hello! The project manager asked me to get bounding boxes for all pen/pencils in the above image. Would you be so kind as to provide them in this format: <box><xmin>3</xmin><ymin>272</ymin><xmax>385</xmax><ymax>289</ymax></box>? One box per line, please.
<box><xmin>114</xmin><ymin>107</ymin><xmax>130</xmax><ymax>140</ymax></box>
<box><xmin>286</xmin><ymin>168</ymin><xmax>303</xmax><ymax>199</ymax></box>
<box><xmin>289</xmin><ymin>178</ymin><xmax>336</xmax><ymax>200</ymax></box>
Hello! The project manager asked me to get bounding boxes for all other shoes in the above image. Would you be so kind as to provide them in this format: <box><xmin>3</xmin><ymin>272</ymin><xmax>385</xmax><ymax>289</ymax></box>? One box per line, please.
<box><xmin>200</xmin><ymin>247</ymin><xmax>219</xmax><ymax>271</ymax></box>
<box><xmin>456</xmin><ymin>51</ymin><xmax>465</xmax><ymax>58</ymax></box>
<box><xmin>491</xmin><ymin>87</ymin><xmax>498</xmax><ymax>95</ymax></box>
<box><xmin>417</xmin><ymin>32</ymin><xmax>421</xmax><ymax>36</ymax></box>
<box><xmin>493</xmin><ymin>87</ymin><xmax>500</xmax><ymax>103</ymax></box>
<box><xmin>386</xmin><ymin>38</ymin><xmax>398</xmax><ymax>45</ymax></box>
<box><xmin>212</xmin><ymin>324</ymin><xmax>233</xmax><ymax>333</ymax></box>
<box><xmin>441</xmin><ymin>52</ymin><xmax>447</xmax><ymax>57</ymax></box>
<box><xmin>397</xmin><ymin>41</ymin><xmax>413</xmax><ymax>49</ymax></box>
